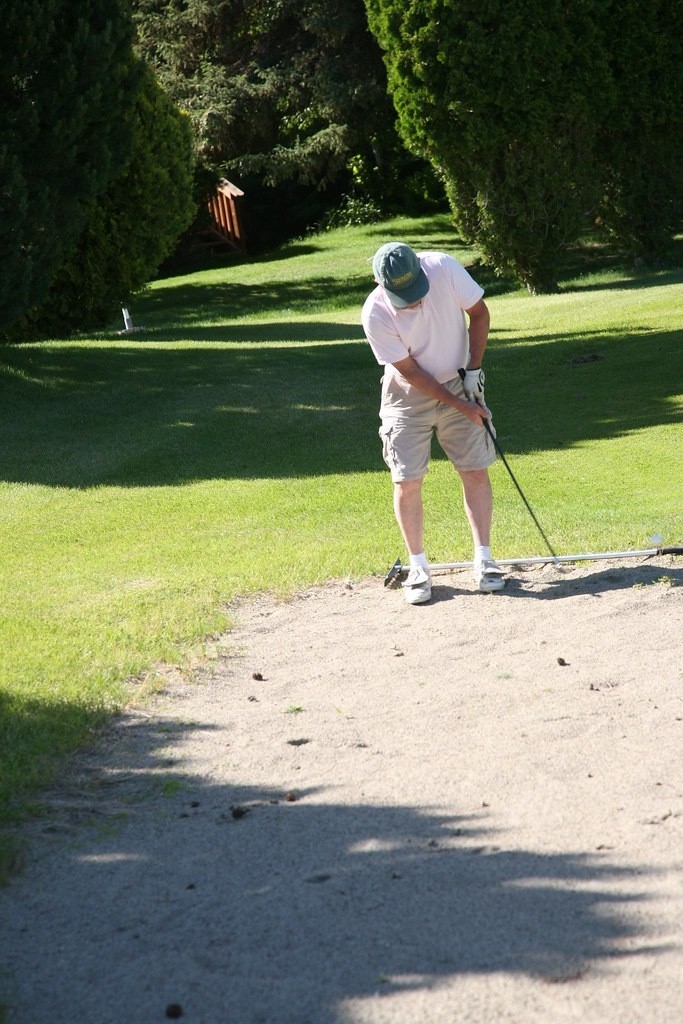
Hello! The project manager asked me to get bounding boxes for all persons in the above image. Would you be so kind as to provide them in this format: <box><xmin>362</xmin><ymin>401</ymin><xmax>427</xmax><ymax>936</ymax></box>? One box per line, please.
<box><xmin>361</xmin><ymin>242</ymin><xmax>507</xmax><ymax>604</ymax></box>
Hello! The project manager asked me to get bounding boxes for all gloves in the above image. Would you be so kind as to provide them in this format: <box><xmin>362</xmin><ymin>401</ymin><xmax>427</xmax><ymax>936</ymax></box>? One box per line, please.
<box><xmin>463</xmin><ymin>367</ymin><xmax>485</xmax><ymax>403</ymax></box>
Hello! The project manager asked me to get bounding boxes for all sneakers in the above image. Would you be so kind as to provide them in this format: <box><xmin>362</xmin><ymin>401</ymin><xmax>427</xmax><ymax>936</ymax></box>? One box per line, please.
<box><xmin>402</xmin><ymin>569</ymin><xmax>434</xmax><ymax>603</ymax></box>
<box><xmin>473</xmin><ymin>558</ymin><xmax>507</xmax><ymax>591</ymax></box>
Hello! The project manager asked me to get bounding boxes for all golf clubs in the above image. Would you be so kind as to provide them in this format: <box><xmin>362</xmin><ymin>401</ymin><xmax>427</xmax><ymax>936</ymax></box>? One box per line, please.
<box><xmin>457</xmin><ymin>367</ymin><xmax>573</xmax><ymax>575</ymax></box>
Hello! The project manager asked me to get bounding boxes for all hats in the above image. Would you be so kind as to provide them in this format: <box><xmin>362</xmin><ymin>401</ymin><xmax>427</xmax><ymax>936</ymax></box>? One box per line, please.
<box><xmin>372</xmin><ymin>242</ymin><xmax>429</xmax><ymax>309</ymax></box>
